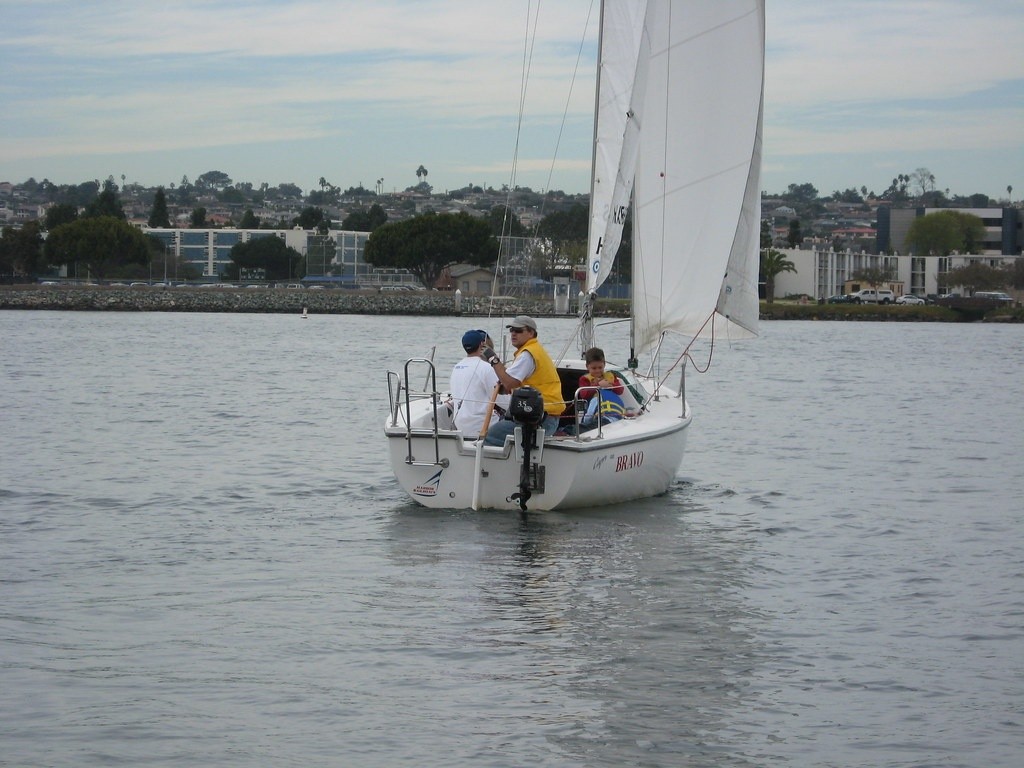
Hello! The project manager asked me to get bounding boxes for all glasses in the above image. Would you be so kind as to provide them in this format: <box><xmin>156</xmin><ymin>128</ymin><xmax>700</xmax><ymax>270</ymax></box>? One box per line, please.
<box><xmin>510</xmin><ymin>328</ymin><xmax>527</xmax><ymax>333</ymax></box>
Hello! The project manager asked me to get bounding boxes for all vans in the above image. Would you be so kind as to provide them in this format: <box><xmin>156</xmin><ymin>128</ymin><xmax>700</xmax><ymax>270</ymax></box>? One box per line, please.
<box><xmin>857</xmin><ymin>289</ymin><xmax>895</xmax><ymax>305</ymax></box>
<box><xmin>975</xmin><ymin>292</ymin><xmax>1014</xmax><ymax>304</ymax></box>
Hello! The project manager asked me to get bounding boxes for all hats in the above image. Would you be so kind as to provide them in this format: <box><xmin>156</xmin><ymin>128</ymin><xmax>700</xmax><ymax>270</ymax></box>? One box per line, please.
<box><xmin>506</xmin><ymin>316</ymin><xmax>537</xmax><ymax>328</ymax></box>
<box><xmin>462</xmin><ymin>330</ymin><xmax>485</xmax><ymax>350</ymax></box>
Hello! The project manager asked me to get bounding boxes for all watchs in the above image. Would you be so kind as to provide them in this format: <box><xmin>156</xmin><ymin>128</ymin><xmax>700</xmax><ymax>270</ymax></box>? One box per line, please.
<box><xmin>490</xmin><ymin>358</ymin><xmax>500</xmax><ymax>366</ymax></box>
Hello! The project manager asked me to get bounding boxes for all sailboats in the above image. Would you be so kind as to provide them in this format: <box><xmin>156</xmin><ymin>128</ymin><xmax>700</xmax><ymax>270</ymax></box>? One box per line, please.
<box><xmin>384</xmin><ymin>0</ymin><xmax>766</xmax><ymax>512</ymax></box>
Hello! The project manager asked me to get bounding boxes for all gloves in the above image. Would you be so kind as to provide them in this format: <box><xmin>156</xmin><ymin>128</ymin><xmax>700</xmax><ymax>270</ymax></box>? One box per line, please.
<box><xmin>479</xmin><ymin>345</ymin><xmax>500</xmax><ymax>365</ymax></box>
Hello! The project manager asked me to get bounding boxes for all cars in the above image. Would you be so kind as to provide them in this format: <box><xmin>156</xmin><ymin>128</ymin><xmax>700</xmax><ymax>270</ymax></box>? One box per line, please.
<box><xmin>825</xmin><ymin>294</ymin><xmax>861</xmax><ymax>305</ymax></box>
<box><xmin>896</xmin><ymin>295</ymin><xmax>925</xmax><ymax>306</ymax></box>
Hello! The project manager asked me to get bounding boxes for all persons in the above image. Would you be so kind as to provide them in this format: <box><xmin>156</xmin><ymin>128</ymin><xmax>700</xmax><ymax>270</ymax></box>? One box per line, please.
<box><xmin>480</xmin><ymin>315</ymin><xmax>566</xmax><ymax>447</ymax></box>
<box><xmin>445</xmin><ymin>330</ymin><xmax>502</xmax><ymax>436</ymax></box>
<box><xmin>578</xmin><ymin>348</ymin><xmax>625</xmax><ymax>423</ymax></box>
